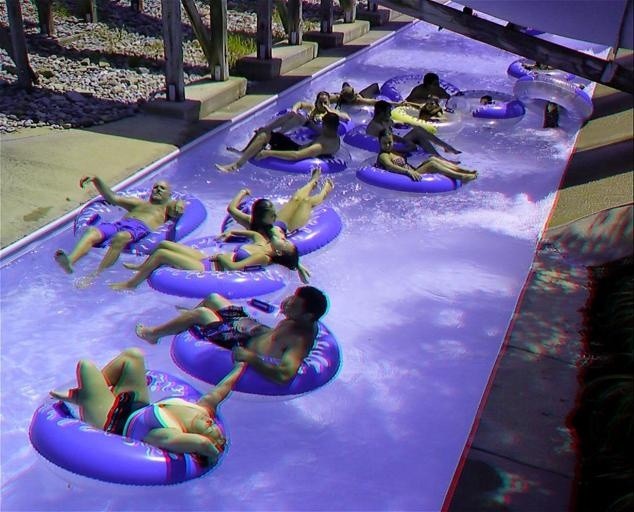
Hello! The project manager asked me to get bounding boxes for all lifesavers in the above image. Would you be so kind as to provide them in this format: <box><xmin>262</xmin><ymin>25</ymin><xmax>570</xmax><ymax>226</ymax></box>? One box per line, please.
<box><xmin>392</xmin><ymin>103</ymin><xmax>463</xmax><ymax>135</ymax></box>
<box><xmin>355</xmin><ymin>153</ymin><xmax>461</xmax><ymax>192</ymax></box>
<box><xmin>447</xmin><ymin>89</ymin><xmax>524</xmax><ymax>127</ymax></box>
<box><xmin>515</xmin><ymin>76</ymin><xmax>593</xmax><ymax>125</ymax></box>
<box><xmin>506</xmin><ymin>58</ymin><xmax>575</xmax><ymax>82</ymax></box>
<box><xmin>149</xmin><ymin>235</ymin><xmax>287</xmax><ymax>297</ymax></box>
<box><xmin>172</xmin><ymin>299</ymin><xmax>342</xmax><ymax>397</ymax></box>
<box><xmin>381</xmin><ymin>75</ymin><xmax>456</xmax><ymax>103</ymax></box>
<box><xmin>73</xmin><ymin>192</ymin><xmax>207</xmax><ymax>255</ymax></box>
<box><xmin>29</xmin><ymin>369</ymin><xmax>224</xmax><ymax>484</ymax></box>
<box><xmin>251</xmin><ymin>145</ymin><xmax>351</xmax><ymax>172</ymax></box>
<box><xmin>340</xmin><ymin>95</ymin><xmax>391</xmax><ymax>117</ymax></box>
<box><xmin>344</xmin><ymin>124</ymin><xmax>410</xmax><ymax>156</ymax></box>
<box><xmin>278</xmin><ymin>107</ymin><xmax>343</xmax><ymax>138</ymax></box>
<box><xmin>224</xmin><ymin>194</ymin><xmax>342</xmax><ymax>255</ymax></box>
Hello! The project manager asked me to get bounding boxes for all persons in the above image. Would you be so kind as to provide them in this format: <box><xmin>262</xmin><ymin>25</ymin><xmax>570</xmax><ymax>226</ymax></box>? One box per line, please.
<box><xmin>437</xmin><ymin>6</ymin><xmax>479</xmax><ymax>33</ymax></box>
<box><xmin>227</xmin><ymin>165</ymin><xmax>334</xmax><ymax>285</ymax></box>
<box><xmin>54</xmin><ymin>174</ymin><xmax>185</xmax><ymax>289</ymax></box>
<box><xmin>253</xmin><ymin>90</ymin><xmax>351</xmax><ymax>136</ymax></box>
<box><xmin>543</xmin><ymin>83</ymin><xmax>585</xmax><ymax>128</ymax></box>
<box><xmin>404</xmin><ymin>73</ymin><xmax>464</xmax><ymax>103</ymax></box>
<box><xmin>107</xmin><ymin>227</ymin><xmax>298</xmax><ymax>293</ymax></box>
<box><xmin>395</xmin><ymin>92</ymin><xmax>449</xmax><ymax>122</ymax></box>
<box><xmin>48</xmin><ymin>346</ymin><xmax>247</xmax><ymax>470</ymax></box>
<box><xmin>366</xmin><ymin>99</ymin><xmax>463</xmax><ymax>165</ymax></box>
<box><xmin>522</xmin><ymin>59</ymin><xmax>549</xmax><ymax>70</ymax></box>
<box><xmin>134</xmin><ymin>284</ymin><xmax>330</xmax><ymax>387</ymax></box>
<box><xmin>479</xmin><ymin>94</ymin><xmax>493</xmax><ymax>105</ymax></box>
<box><xmin>214</xmin><ymin>112</ymin><xmax>341</xmax><ymax>173</ymax></box>
<box><xmin>378</xmin><ymin>128</ymin><xmax>478</xmax><ymax>185</ymax></box>
<box><xmin>330</xmin><ymin>82</ymin><xmax>408</xmax><ymax>111</ymax></box>
